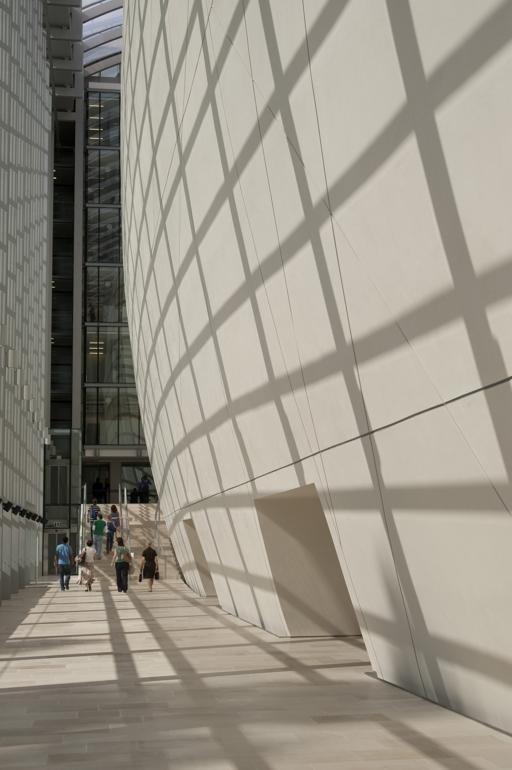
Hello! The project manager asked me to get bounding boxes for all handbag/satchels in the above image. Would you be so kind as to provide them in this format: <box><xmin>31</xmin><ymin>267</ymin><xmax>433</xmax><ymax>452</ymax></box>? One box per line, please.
<box><xmin>138</xmin><ymin>574</ymin><xmax>143</xmax><ymax>582</ymax></box>
<box><xmin>155</xmin><ymin>571</ymin><xmax>159</xmax><ymax>580</ymax></box>
<box><xmin>120</xmin><ymin>552</ymin><xmax>132</xmax><ymax>563</ymax></box>
<box><xmin>107</xmin><ymin>523</ymin><xmax>116</xmax><ymax>532</ymax></box>
<box><xmin>77</xmin><ymin>557</ymin><xmax>85</xmax><ymax>565</ymax></box>
<box><xmin>115</xmin><ymin>519</ymin><xmax>121</xmax><ymax>528</ymax></box>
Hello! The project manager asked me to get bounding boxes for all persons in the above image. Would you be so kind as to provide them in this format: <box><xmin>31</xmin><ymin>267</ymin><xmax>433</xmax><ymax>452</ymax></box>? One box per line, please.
<box><xmin>139</xmin><ymin>539</ymin><xmax>160</xmax><ymax>592</ymax></box>
<box><xmin>104</xmin><ymin>513</ymin><xmax>121</xmax><ymax>555</ymax></box>
<box><xmin>75</xmin><ymin>540</ymin><xmax>97</xmax><ymax>592</ymax></box>
<box><xmin>111</xmin><ymin>537</ymin><xmax>133</xmax><ymax>593</ymax></box>
<box><xmin>87</xmin><ymin>499</ymin><xmax>102</xmax><ymax>542</ymax></box>
<box><xmin>54</xmin><ymin>536</ymin><xmax>74</xmax><ymax>591</ymax></box>
<box><xmin>139</xmin><ymin>474</ymin><xmax>153</xmax><ymax>503</ymax></box>
<box><xmin>92</xmin><ymin>474</ymin><xmax>104</xmax><ymax>504</ymax></box>
<box><xmin>110</xmin><ymin>505</ymin><xmax>122</xmax><ymax>544</ymax></box>
<box><xmin>92</xmin><ymin>513</ymin><xmax>106</xmax><ymax>560</ymax></box>
<box><xmin>130</xmin><ymin>487</ymin><xmax>139</xmax><ymax>503</ymax></box>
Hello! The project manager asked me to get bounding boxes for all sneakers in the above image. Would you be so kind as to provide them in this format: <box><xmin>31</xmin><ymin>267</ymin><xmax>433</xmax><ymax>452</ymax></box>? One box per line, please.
<box><xmin>106</xmin><ymin>550</ymin><xmax>112</xmax><ymax>555</ymax></box>
<box><xmin>96</xmin><ymin>556</ymin><xmax>101</xmax><ymax>561</ymax></box>
